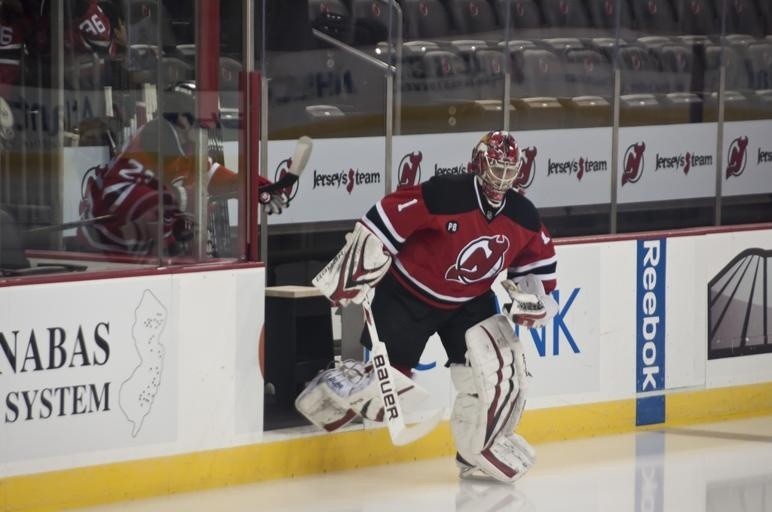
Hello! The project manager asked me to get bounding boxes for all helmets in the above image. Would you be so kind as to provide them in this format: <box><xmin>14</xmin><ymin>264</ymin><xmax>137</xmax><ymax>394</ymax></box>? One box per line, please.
<box><xmin>471</xmin><ymin>129</ymin><xmax>523</xmax><ymax>205</ymax></box>
<box><xmin>159</xmin><ymin>80</ymin><xmax>222</xmax><ymax>132</ymax></box>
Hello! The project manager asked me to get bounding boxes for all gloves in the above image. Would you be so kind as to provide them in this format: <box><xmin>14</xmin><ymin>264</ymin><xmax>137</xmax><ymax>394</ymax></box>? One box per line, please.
<box><xmin>500</xmin><ymin>274</ymin><xmax>559</xmax><ymax>329</ymax></box>
<box><xmin>228</xmin><ymin>172</ymin><xmax>295</xmax><ymax>215</ymax></box>
<box><xmin>163</xmin><ymin>182</ymin><xmax>219</xmax><ymax>219</ymax></box>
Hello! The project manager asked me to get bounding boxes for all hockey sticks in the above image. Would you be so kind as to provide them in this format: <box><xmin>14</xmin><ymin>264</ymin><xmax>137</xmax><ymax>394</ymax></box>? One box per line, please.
<box><xmin>359</xmin><ymin>291</ymin><xmax>446</xmax><ymax>450</ymax></box>
<box><xmin>21</xmin><ymin>135</ymin><xmax>313</xmax><ymax>239</ymax></box>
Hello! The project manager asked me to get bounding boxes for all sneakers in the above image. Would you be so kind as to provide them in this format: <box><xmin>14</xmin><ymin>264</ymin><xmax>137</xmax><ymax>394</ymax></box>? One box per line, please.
<box><xmin>455</xmin><ymin>451</ymin><xmax>503</xmax><ymax>477</ymax></box>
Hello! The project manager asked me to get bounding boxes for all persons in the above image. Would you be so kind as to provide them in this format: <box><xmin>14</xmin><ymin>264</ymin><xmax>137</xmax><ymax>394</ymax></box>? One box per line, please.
<box><xmin>1</xmin><ymin>0</ymin><xmax>119</xmax><ymax>132</ymax></box>
<box><xmin>291</xmin><ymin>131</ymin><xmax>558</xmax><ymax>485</ymax></box>
<box><xmin>77</xmin><ymin>80</ymin><xmax>289</xmax><ymax>265</ymax></box>
